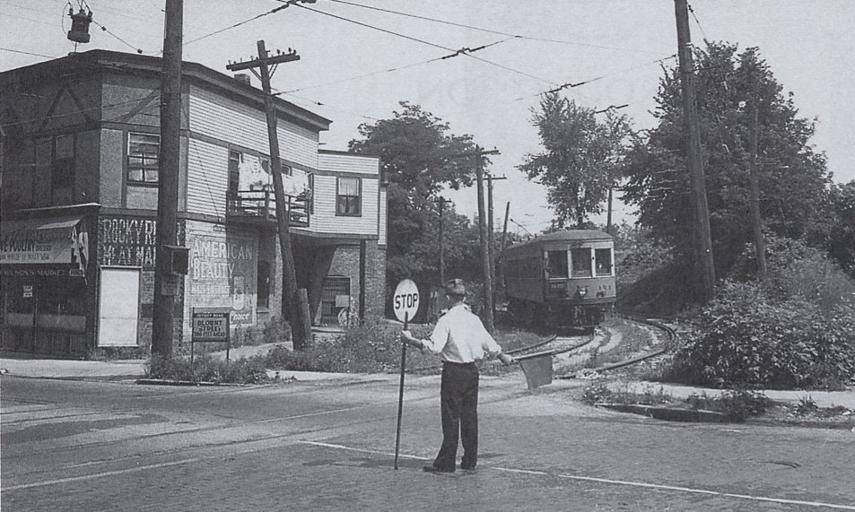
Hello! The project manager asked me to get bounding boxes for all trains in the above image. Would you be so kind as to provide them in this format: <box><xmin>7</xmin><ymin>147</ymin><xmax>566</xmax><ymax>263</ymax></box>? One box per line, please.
<box><xmin>501</xmin><ymin>228</ymin><xmax>617</xmax><ymax>335</ymax></box>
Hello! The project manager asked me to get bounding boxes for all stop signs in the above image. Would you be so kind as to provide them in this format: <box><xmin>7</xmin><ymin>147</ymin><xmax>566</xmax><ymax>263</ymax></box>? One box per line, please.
<box><xmin>392</xmin><ymin>278</ymin><xmax>421</xmax><ymax>323</ymax></box>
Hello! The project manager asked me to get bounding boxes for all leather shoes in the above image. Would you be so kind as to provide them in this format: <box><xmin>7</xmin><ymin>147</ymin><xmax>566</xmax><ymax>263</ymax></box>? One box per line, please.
<box><xmin>422</xmin><ymin>465</ymin><xmax>454</xmax><ymax>472</ymax></box>
<box><xmin>460</xmin><ymin>462</ymin><xmax>475</xmax><ymax>469</ymax></box>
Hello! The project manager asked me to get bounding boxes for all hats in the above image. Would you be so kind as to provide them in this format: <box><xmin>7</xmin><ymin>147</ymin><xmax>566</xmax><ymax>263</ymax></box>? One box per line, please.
<box><xmin>444</xmin><ymin>278</ymin><xmax>468</xmax><ymax>295</ymax></box>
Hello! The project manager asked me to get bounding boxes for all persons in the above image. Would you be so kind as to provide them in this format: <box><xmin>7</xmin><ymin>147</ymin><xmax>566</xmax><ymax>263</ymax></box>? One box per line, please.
<box><xmin>398</xmin><ymin>279</ymin><xmax>518</xmax><ymax>476</ymax></box>
